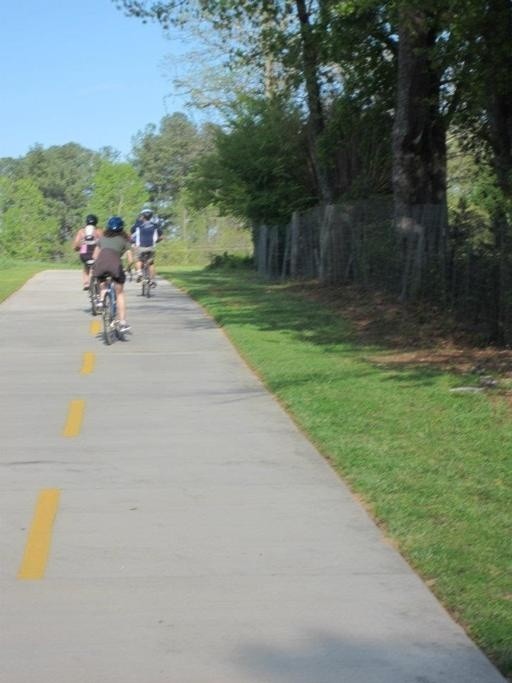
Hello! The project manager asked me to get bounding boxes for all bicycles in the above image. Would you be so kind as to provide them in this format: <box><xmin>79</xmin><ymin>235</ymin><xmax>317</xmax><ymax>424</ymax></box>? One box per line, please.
<box><xmin>139</xmin><ymin>248</ymin><xmax>156</xmax><ymax>298</ymax></box>
<box><xmin>85</xmin><ymin>259</ymin><xmax>132</xmax><ymax>345</ymax></box>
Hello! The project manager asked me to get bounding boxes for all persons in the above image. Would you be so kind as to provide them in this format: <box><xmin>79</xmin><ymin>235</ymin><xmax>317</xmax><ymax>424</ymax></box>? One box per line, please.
<box><xmin>126</xmin><ymin>219</ymin><xmax>144</xmax><ymax>272</ymax></box>
<box><xmin>130</xmin><ymin>206</ymin><xmax>164</xmax><ymax>290</ymax></box>
<box><xmin>72</xmin><ymin>213</ymin><xmax>102</xmax><ymax>290</ymax></box>
<box><xmin>91</xmin><ymin>216</ymin><xmax>133</xmax><ymax>332</ymax></box>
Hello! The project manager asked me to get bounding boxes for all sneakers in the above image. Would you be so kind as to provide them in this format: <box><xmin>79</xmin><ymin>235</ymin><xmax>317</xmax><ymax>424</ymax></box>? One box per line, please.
<box><xmin>83</xmin><ymin>271</ymin><xmax>157</xmax><ymax>333</ymax></box>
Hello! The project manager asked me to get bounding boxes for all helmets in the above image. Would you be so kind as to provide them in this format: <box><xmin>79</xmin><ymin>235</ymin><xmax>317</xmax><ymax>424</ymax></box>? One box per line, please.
<box><xmin>106</xmin><ymin>216</ymin><xmax>124</xmax><ymax>230</ymax></box>
<box><xmin>86</xmin><ymin>215</ymin><xmax>98</xmax><ymax>225</ymax></box>
<box><xmin>139</xmin><ymin>208</ymin><xmax>154</xmax><ymax>219</ymax></box>
